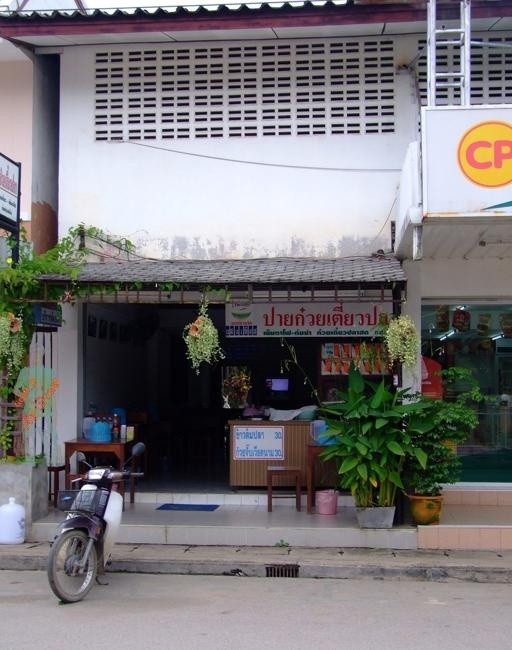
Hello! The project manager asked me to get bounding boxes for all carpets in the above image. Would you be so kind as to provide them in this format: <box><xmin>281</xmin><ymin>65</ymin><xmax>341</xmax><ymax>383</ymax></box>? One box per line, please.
<box><xmin>156</xmin><ymin>503</ymin><xmax>220</xmax><ymax>512</ymax></box>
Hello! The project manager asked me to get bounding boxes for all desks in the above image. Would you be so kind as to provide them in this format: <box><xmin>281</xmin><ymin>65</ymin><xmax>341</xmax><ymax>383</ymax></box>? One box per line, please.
<box><xmin>64</xmin><ymin>438</ymin><xmax>138</xmax><ymax>513</ymax></box>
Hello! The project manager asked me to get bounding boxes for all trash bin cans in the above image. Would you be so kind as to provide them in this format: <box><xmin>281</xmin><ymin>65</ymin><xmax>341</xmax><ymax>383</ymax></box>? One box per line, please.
<box><xmin>315</xmin><ymin>490</ymin><xmax>337</xmax><ymax>515</ymax></box>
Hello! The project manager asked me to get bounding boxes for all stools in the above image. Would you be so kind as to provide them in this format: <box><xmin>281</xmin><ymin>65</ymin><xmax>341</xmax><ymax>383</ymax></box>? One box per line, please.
<box><xmin>47</xmin><ymin>465</ymin><xmax>65</xmax><ymax>507</ymax></box>
<box><xmin>265</xmin><ymin>464</ymin><xmax>303</xmax><ymax>513</ymax></box>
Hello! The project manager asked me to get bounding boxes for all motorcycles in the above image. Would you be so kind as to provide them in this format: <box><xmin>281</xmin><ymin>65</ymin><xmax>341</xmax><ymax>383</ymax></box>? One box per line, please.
<box><xmin>48</xmin><ymin>440</ymin><xmax>146</xmax><ymax>604</ymax></box>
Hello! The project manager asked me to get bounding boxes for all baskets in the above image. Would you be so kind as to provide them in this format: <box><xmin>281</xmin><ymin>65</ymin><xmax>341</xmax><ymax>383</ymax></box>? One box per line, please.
<box><xmin>59</xmin><ymin>489</ymin><xmax>110</xmax><ymax>516</ymax></box>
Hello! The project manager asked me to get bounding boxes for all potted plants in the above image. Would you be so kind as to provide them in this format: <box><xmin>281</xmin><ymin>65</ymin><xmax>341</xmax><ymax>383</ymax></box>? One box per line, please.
<box><xmin>310</xmin><ymin>360</ymin><xmax>504</xmax><ymax>532</ymax></box>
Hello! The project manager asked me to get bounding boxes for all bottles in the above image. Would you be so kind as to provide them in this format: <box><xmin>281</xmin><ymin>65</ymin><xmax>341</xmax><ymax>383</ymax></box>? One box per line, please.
<box><xmin>120</xmin><ymin>424</ymin><xmax>126</xmax><ymax>439</ymax></box>
<box><xmin>83</xmin><ymin>411</ymin><xmax>120</xmax><ymax>434</ymax></box>
